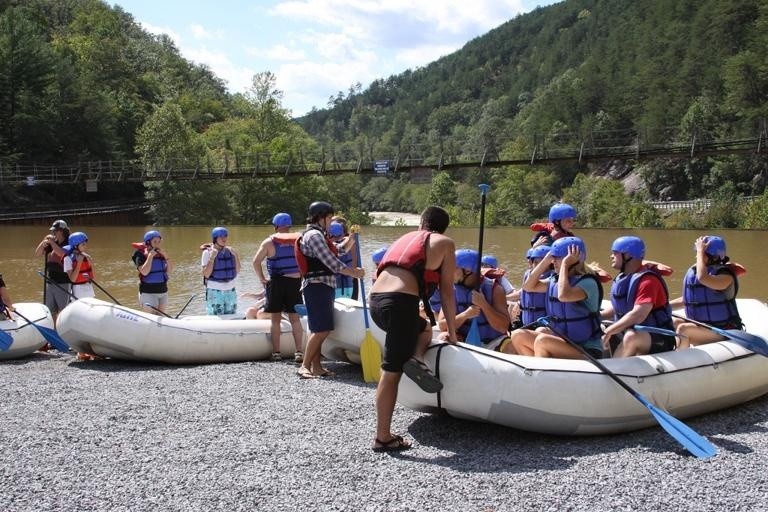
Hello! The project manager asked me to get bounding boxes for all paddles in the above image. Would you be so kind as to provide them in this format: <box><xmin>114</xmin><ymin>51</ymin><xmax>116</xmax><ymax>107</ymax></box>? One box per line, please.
<box><xmin>0</xmin><ymin>302</ymin><xmax>70</xmax><ymax>354</ymax></box>
<box><xmin>601</xmin><ymin>318</ymin><xmax>686</xmax><ymax>340</ymax></box>
<box><xmin>465</xmin><ymin>183</ymin><xmax>489</xmax><ymax>347</ymax></box>
<box><xmin>673</xmin><ymin>311</ymin><xmax>768</xmax><ymax>356</ymax></box>
<box><xmin>351</xmin><ymin>225</ymin><xmax>382</xmax><ymax>384</ymax></box>
<box><xmin>539</xmin><ymin>316</ymin><xmax>718</xmax><ymax>459</ymax></box>
<box><xmin>0</xmin><ymin>330</ymin><xmax>13</xmax><ymax>350</ymax></box>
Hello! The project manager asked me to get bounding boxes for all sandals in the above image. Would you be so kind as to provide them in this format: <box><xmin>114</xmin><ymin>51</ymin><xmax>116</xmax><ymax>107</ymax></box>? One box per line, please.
<box><xmin>371</xmin><ymin>433</ymin><xmax>414</xmax><ymax>451</ymax></box>
<box><xmin>404</xmin><ymin>357</ymin><xmax>444</xmax><ymax>394</ymax></box>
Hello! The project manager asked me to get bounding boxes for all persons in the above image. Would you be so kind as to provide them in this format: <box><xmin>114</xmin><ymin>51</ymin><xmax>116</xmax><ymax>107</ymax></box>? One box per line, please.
<box><xmin>131</xmin><ymin>230</ymin><xmax>173</xmax><ymax>316</ymax></box>
<box><xmin>60</xmin><ymin>231</ymin><xmax>95</xmax><ymax>359</ymax></box>
<box><xmin>251</xmin><ymin>212</ymin><xmax>305</xmax><ymax>363</ymax></box>
<box><xmin>200</xmin><ymin>226</ymin><xmax>241</xmax><ymax>316</ymax></box>
<box><xmin>0</xmin><ymin>273</ymin><xmax>16</xmax><ymax>320</ymax></box>
<box><xmin>366</xmin><ymin>202</ymin><xmax>457</xmax><ymax>454</ymax></box>
<box><xmin>371</xmin><ymin>246</ymin><xmax>388</xmax><ymax>285</ymax></box>
<box><xmin>422</xmin><ymin>202</ymin><xmax>746</xmax><ymax>360</ymax></box>
<box><xmin>293</xmin><ymin>200</ymin><xmax>365</xmax><ymax>380</ymax></box>
<box><xmin>34</xmin><ymin>219</ymin><xmax>72</xmax><ymax>352</ymax></box>
<box><xmin>239</xmin><ymin>288</ymin><xmax>273</xmax><ymax>320</ymax></box>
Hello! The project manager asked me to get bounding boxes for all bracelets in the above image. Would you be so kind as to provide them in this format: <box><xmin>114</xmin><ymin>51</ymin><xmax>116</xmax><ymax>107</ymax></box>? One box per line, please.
<box><xmin>209</xmin><ymin>259</ymin><xmax>214</xmax><ymax>263</ymax></box>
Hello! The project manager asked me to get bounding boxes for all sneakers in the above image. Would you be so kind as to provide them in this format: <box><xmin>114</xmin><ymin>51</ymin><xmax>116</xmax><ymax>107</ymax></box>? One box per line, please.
<box><xmin>293</xmin><ymin>351</ymin><xmax>305</xmax><ymax>362</ymax></box>
<box><xmin>268</xmin><ymin>351</ymin><xmax>282</xmax><ymax>361</ymax></box>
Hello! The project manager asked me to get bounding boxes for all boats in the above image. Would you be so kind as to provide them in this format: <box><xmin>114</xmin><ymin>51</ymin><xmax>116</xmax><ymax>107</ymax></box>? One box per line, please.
<box><xmin>381</xmin><ymin>299</ymin><xmax>763</xmax><ymax>441</ymax></box>
<box><xmin>322</xmin><ymin>295</ymin><xmax>426</xmax><ymax>362</ymax></box>
<box><xmin>56</xmin><ymin>298</ymin><xmax>322</xmax><ymax>363</ymax></box>
<box><xmin>0</xmin><ymin>303</ymin><xmax>52</xmax><ymax>361</ymax></box>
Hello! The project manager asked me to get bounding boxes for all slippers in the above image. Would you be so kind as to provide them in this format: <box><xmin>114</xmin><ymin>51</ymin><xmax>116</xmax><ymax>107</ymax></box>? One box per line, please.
<box><xmin>312</xmin><ymin>368</ymin><xmax>337</xmax><ymax>377</ymax></box>
<box><xmin>295</xmin><ymin>367</ymin><xmax>316</xmax><ymax>379</ymax></box>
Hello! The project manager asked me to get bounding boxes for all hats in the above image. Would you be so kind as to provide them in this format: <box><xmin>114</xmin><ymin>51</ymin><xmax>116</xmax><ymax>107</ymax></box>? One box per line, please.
<box><xmin>48</xmin><ymin>220</ymin><xmax>69</xmax><ymax>232</ymax></box>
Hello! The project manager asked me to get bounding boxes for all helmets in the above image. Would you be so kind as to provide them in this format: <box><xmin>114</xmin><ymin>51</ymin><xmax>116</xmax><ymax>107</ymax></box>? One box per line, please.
<box><xmin>531</xmin><ymin>246</ymin><xmax>551</xmax><ymax>258</ymax></box>
<box><xmin>372</xmin><ymin>248</ymin><xmax>387</xmax><ymax>263</ymax></box>
<box><xmin>549</xmin><ymin>203</ymin><xmax>577</xmax><ymax>220</ymax></box>
<box><xmin>454</xmin><ymin>250</ymin><xmax>480</xmax><ymax>273</ymax></box>
<box><xmin>307</xmin><ymin>201</ymin><xmax>332</xmax><ymax>218</ymax></box>
<box><xmin>67</xmin><ymin>232</ymin><xmax>89</xmax><ymax>249</ymax></box>
<box><xmin>480</xmin><ymin>255</ymin><xmax>497</xmax><ymax>268</ymax></box>
<box><xmin>211</xmin><ymin>226</ymin><xmax>229</xmax><ymax>238</ymax></box>
<box><xmin>611</xmin><ymin>236</ymin><xmax>645</xmax><ymax>259</ymax></box>
<box><xmin>271</xmin><ymin>212</ymin><xmax>294</xmax><ymax>227</ymax></box>
<box><xmin>143</xmin><ymin>230</ymin><xmax>162</xmax><ymax>242</ymax></box>
<box><xmin>694</xmin><ymin>235</ymin><xmax>726</xmax><ymax>260</ymax></box>
<box><xmin>327</xmin><ymin>221</ymin><xmax>345</xmax><ymax>236</ymax></box>
<box><xmin>550</xmin><ymin>236</ymin><xmax>585</xmax><ymax>258</ymax></box>
<box><xmin>525</xmin><ymin>247</ymin><xmax>533</xmax><ymax>258</ymax></box>
